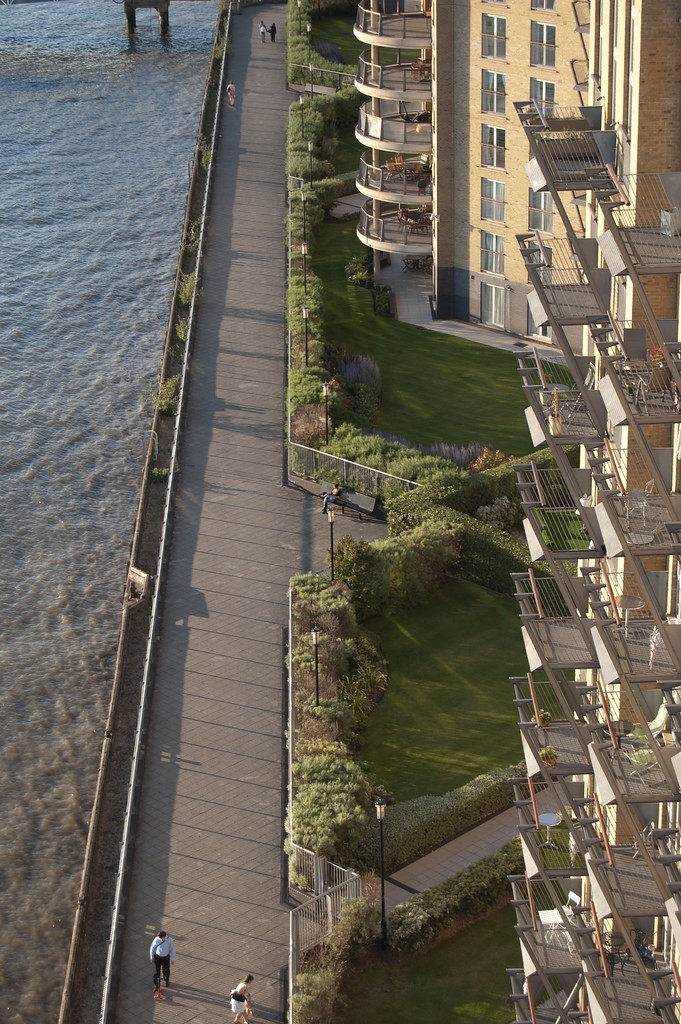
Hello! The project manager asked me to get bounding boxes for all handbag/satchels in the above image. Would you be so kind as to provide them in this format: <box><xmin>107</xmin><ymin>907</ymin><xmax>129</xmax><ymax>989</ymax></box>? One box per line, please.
<box><xmin>232</xmin><ymin>991</ymin><xmax>247</xmax><ymax>1002</ymax></box>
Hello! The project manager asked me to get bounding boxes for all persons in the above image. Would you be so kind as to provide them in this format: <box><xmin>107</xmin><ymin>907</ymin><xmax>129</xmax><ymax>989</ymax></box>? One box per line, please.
<box><xmin>230</xmin><ymin>974</ymin><xmax>254</xmax><ymax>1024</ymax></box>
<box><xmin>259</xmin><ymin>21</ymin><xmax>276</xmax><ymax>43</ymax></box>
<box><xmin>227</xmin><ymin>81</ymin><xmax>235</xmax><ymax>106</ymax></box>
<box><xmin>150</xmin><ymin>931</ymin><xmax>176</xmax><ymax>987</ymax></box>
<box><xmin>322</xmin><ymin>484</ymin><xmax>341</xmax><ymax>514</ymax></box>
<box><xmin>245</xmin><ymin>993</ymin><xmax>255</xmax><ymax>1015</ymax></box>
